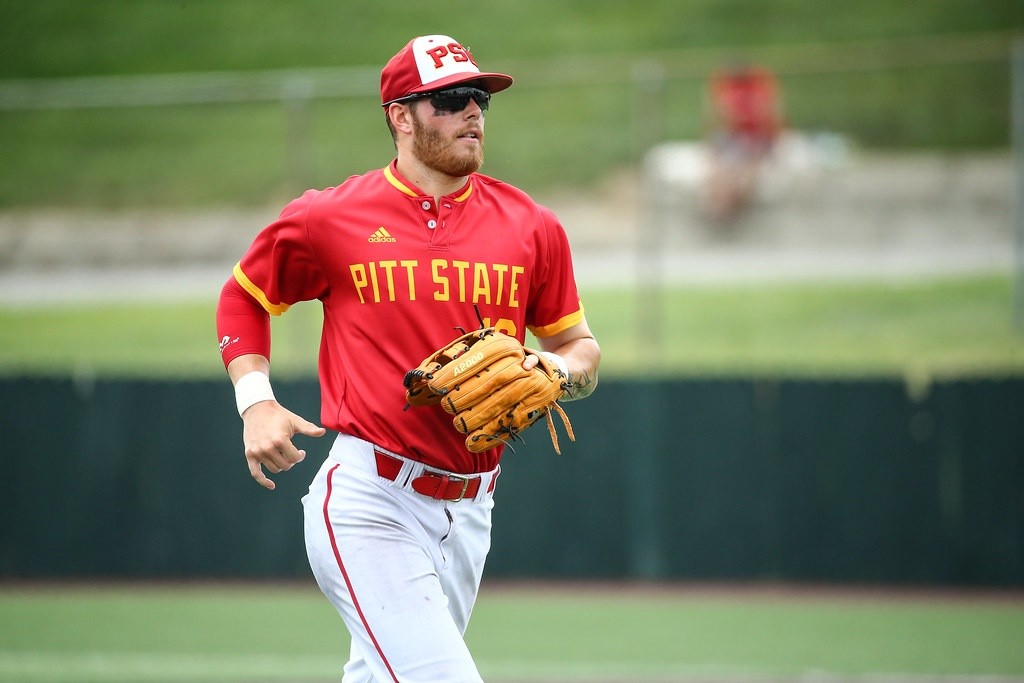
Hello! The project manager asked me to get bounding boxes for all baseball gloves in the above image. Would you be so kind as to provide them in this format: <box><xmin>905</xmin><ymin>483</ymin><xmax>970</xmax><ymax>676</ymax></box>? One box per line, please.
<box><xmin>402</xmin><ymin>303</ymin><xmax>577</xmax><ymax>457</ymax></box>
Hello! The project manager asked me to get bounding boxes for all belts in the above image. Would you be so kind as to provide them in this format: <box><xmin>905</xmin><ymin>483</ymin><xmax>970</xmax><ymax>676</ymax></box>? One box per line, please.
<box><xmin>374</xmin><ymin>449</ymin><xmax>499</xmax><ymax>502</ymax></box>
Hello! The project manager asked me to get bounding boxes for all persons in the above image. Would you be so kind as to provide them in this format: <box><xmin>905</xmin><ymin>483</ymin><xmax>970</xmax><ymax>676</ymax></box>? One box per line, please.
<box><xmin>217</xmin><ymin>35</ymin><xmax>601</xmax><ymax>683</ymax></box>
<box><xmin>708</xmin><ymin>47</ymin><xmax>777</xmax><ymax>214</ymax></box>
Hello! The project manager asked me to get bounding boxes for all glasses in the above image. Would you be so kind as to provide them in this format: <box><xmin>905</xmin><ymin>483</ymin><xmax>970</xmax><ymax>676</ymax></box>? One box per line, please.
<box><xmin>382</xmin><ymin>84</ymin><xmax>491</xmax><ymax>111</ymax></box>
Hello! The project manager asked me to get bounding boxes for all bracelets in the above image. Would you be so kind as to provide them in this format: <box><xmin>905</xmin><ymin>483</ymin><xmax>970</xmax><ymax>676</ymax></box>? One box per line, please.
<box><xmin>232</xmin><ymin>372</ymin><xmax>275</xmax><ymax>417</ymax></box>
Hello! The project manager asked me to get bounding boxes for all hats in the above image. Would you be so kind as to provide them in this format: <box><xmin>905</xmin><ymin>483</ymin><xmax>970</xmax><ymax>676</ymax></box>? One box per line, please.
<box><xmin>380</xmin><ymin>35</ymin><xmax>514</xmax><ymax>116</ymax></box>
